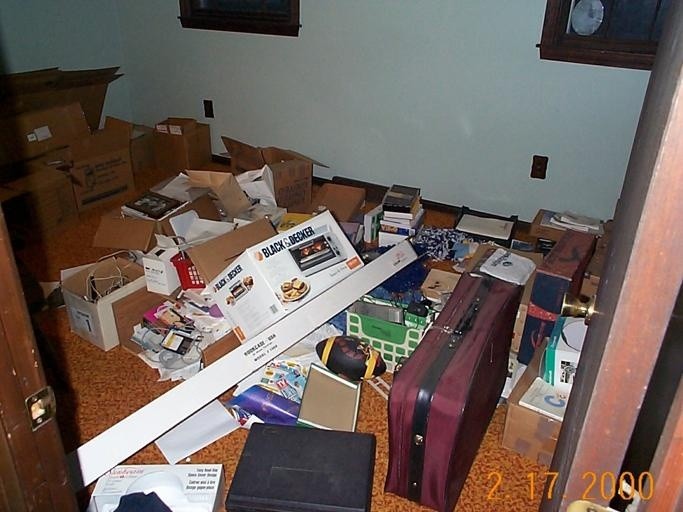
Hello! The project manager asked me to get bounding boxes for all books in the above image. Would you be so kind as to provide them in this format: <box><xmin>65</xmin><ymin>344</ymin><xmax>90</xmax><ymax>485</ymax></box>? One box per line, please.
<box><xmin>540</xmin><ymin>209</ymin><xmax>601</xmax><ymax>233</ymax></box>
<box><xmin>234</xmin><ymin>184</ymin><xmax>426</xmax><ymax>249</ymax></box>
<box><xmin>120</xmin><ymin>190</ymin><xmax>182</xmax><ymax>223</ymax></box>
<box><xmin>518</xmin><ymin>376</ymin><xmax>571</xmax><ymax>422</ymax></box>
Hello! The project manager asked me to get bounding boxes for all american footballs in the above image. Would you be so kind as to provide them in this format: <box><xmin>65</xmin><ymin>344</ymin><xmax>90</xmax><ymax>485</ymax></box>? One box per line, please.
<box><xmin>314</xmin><ymin>335</ymin><xmax>385</xmax><ymax>381</ymax></box>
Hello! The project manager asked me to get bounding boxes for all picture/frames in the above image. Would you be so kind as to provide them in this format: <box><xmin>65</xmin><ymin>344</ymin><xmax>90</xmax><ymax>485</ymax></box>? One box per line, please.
<box><xmin>536</xmin><ymin>0</ymin><xmax>669</xmax><ymax>71</ymax></box>
<box><xmin>176</xmin><ymin>1</ymin><xmax>302</xmax><ymax>36</ymax></box>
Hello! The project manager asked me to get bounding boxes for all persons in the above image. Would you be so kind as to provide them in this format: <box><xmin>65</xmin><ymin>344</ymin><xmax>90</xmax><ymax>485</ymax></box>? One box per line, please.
<box><xmin>293</xmin><ymin>370</ymin><xmax>302</xmax><ymax>379</ymax></box>
<box><xmin>284</xmin><ymin>379</ymin><xmax>303</xmax><ymax>402</ymax></box>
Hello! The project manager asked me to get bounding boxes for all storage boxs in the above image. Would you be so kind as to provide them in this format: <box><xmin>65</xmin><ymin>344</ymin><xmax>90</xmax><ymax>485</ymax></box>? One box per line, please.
<box><xmin>500</xmin><ymin>338</ymin><xmax>563</xmax><ymax>468</ymax></box>
<box><xmin>530</xmin><ymin>209</ymin><xmax>569</xmax><ymax>243</ymax></box>
<box><xmin>517</xmin><ymin>229</ymin><xmax>597</xmax><ymax>367</ymax></box>
<box><xmin>348</xmin><ymin>295</ymin><xmax>433</xmax><ymax>376</ymax></box>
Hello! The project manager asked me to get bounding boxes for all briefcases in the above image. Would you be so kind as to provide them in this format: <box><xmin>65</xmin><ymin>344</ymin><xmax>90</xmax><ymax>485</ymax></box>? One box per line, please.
<box><xmin>383</xmin><ymin>271</ymin><xmax>524</xmax><ymax>507</ymax></box>
